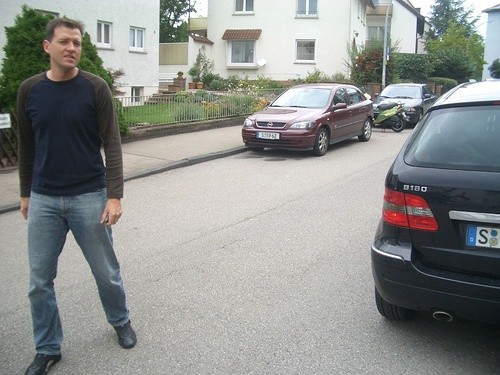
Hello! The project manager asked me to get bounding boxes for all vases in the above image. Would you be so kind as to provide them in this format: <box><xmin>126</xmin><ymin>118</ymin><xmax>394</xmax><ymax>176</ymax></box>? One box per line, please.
<box><xmin>196</xmin><ymin>81</ymin><xmax>204</xmax><ymax>89</ymax></box>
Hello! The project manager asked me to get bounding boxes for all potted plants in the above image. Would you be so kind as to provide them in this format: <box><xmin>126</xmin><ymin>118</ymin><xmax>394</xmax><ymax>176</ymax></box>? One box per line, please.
<box><xmin>187</xmin><ymin>66</ymin><xmax>199</xmax><ymax>89</ymax></box>
<box><xmin>177</xmin><ymin>71</ymin><xmax>184</xmax><ymax>78</ymax></box>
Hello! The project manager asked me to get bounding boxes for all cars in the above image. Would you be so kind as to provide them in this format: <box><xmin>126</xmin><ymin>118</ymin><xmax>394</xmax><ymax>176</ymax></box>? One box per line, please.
<box><xmin>240</xmin><ymin>81</ymin><xmax>374</xmax><ymax>155</ymax></box>
<box><xmin>371</xmin><ymin>80</ymin><xmax>500</xmax><ymax>325</ymax></box>
<box><xmin>373</xmin><ymin>83</ymin><xmax>438</xmax><ymax>126</ymax></box>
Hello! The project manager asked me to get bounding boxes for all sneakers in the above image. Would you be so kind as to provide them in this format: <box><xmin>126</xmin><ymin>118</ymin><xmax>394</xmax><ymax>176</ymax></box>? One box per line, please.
<box><xmin>114</xmin><ymin>320</ymin><xmax>137</xmax><ymax>348</ymax></box>
<box><xmin>24</xmin><ymin>353</ymin><xmax>62</xmax><ymax>375</ymax></box>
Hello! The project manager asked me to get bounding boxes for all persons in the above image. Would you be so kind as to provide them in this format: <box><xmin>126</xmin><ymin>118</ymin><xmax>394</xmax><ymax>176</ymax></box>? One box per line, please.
<box><xmin>16</xmin><ymin>17</ymin><xmax>137</xmax><ymax>375</ymax></box>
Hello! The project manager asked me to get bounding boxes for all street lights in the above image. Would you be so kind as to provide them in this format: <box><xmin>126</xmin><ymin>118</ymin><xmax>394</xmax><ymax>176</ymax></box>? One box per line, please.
<box><xmin>467</xmin><ymin>20</ymin><xmax>496</xmax><ymax>56</ymax></box>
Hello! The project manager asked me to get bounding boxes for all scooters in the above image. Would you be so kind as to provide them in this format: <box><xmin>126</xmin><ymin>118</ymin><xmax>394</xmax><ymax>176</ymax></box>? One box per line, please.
<box><xmin>362</xmin><ymin>86</ymin><xmax>410</xmax><ymax>132</ymax></box>
